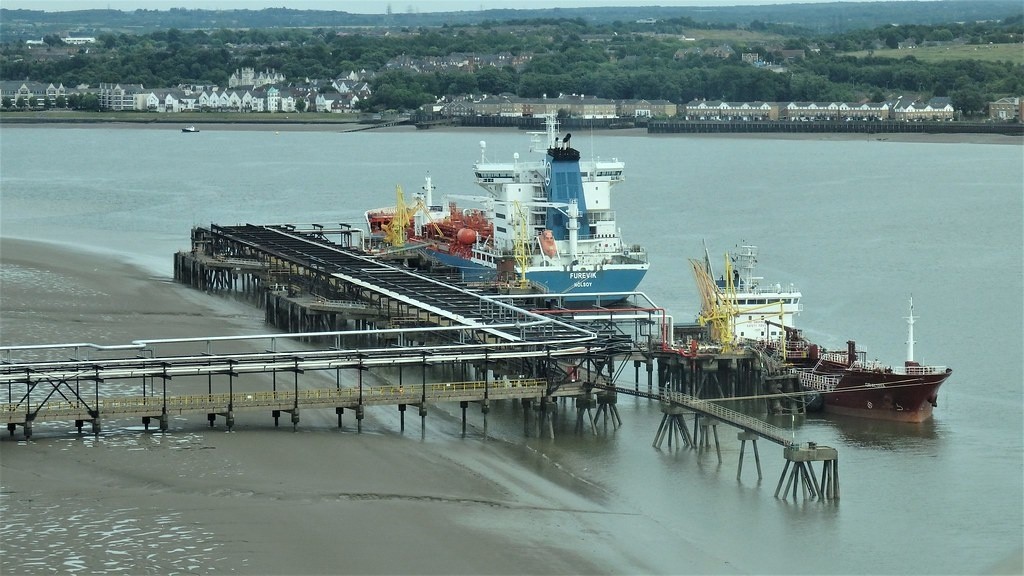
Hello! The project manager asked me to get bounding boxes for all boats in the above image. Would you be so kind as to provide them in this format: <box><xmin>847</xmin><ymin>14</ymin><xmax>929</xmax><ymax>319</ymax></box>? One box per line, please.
<box><xmin>540</xmin><ymin>230</ymin><xmax>557</xmax><ymax>257</ymax></box>
<box><xmin>181</xmin><ymin>127</ymin><xmax>200</xmax><ymax>132</ymax></box>
<box><xmin>364</xmin><ymin>107</ymin><xmax>651</xmax><ymax>311</ymax></box>
<box><xmin>660</xmin><ymin>237</ymin><xmax>954</xmax><ymax>423</ymax></box>
